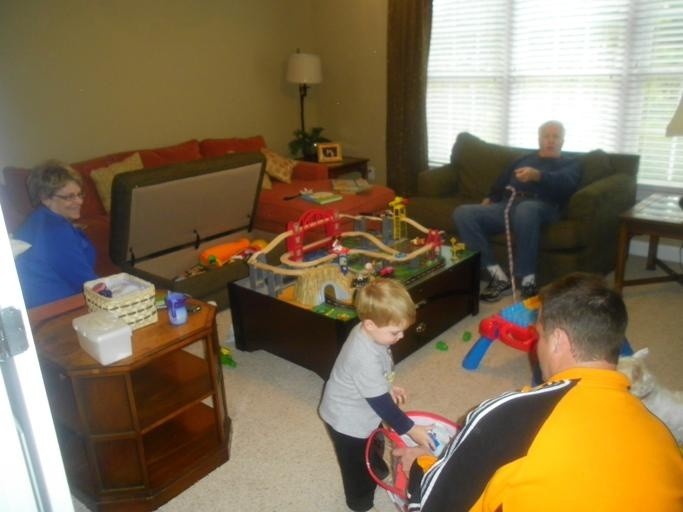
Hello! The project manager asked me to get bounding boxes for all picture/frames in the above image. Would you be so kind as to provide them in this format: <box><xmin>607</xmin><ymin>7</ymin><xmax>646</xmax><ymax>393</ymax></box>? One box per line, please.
<box><xmin>316</xmin><ymin>142</ymin><xmax>342</xmax><ymax>163</ymax></box>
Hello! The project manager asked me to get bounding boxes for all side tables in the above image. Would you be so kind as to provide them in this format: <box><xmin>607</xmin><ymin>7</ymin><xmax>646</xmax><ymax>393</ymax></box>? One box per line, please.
<box><xmin>306</xmin><ymin>153</ymin><xmax>372</xmax><ymax>188</ymax></box>
<box><xmin>613</xmin><ymin>193</ymin><xmax>683</xmax><ymax>313</ymax></box>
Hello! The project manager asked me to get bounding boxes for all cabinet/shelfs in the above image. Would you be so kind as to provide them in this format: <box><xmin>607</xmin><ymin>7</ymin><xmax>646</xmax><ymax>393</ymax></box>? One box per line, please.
<box><xmin>33</xmin><ymin>289</ymin><xmax>230</xmax><ymax>512</ymax></box>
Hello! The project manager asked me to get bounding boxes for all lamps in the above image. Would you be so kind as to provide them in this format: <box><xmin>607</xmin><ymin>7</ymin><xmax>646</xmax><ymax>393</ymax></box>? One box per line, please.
<box><xmin>664</xmin><ymin>96</ymin><xmax>683</xmax><ymax>210</ymax></box>
<box><xmin>287</xmin><ymin>48</ymin><xmax>330</xmax><ymax>156</ymax></box>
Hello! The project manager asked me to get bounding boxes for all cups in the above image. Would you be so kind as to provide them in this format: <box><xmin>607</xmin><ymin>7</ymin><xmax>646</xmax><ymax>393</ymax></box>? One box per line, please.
<box><xmin>164</xmin><ymin>293</ymin><xmax>186</xmax><ymax>325</ymax></box>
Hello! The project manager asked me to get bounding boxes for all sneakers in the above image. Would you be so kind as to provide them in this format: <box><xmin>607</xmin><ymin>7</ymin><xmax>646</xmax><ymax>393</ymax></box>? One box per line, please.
<box><xmin>480</xmin><ymin>272</ymin><xmax>513</xmax><ymax>303</ymax></box>
<box><xmin>520</xmin><ymin>280</ymin><xmax>538</xmax><ymax>297</ymax></box>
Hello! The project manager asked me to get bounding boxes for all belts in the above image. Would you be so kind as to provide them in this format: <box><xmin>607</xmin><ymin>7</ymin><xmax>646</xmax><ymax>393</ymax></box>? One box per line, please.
<box><xmin>504</xmin><ymin>192</ymin><xmax>532</xmax><ymax>198</ymax></box>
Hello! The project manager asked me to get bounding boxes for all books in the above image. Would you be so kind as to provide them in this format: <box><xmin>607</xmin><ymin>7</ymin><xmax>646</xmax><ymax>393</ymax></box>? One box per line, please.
<box><xmin>298</xmin><ymin>176</ymin><xmax>375</xmax><ymax>206</ymax></box>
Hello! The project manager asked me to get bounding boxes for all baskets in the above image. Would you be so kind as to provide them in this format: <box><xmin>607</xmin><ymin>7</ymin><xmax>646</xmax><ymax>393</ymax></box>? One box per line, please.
<box><xmin>83</xmin><ymin>272</ymin><xmax>158</xmax><ymax>332</ymax></box>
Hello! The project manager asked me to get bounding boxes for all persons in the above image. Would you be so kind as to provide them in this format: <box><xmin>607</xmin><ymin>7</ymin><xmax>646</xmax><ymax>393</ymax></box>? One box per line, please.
<box><xmin>314</xmin><ymin>277</ymin><xmax>438</xmax><ymax>511</ymax></box>
<box><xmin>11</xmin><ymin>155</ymin><xmax>99</xmax><ymax>310</ymax></box>
<box><xmin>443</xmin><ymin>120</ymin><xmax>582</xmax><ymax>303</ymax></box>
<box><xmin>391</xmin><ymin>269</ymin><xmax>683</xmax><ymax>512</ymax></box>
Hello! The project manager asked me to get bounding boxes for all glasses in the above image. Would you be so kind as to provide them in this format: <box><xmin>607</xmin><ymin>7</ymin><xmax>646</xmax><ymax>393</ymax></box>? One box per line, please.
<box><xmin>51</xmin><ymin>191</ymin><xmax>84</xmax><ymax>202</ymax></box>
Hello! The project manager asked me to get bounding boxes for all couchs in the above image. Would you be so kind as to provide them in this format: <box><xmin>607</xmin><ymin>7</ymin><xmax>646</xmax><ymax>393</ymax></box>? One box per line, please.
<box><xmin>407</xmin><ymin>126</ymin><xmax>643</xmax><ymax>296</ymax></box>
<box><xmin>0</xmin><ymin>134</ymin><xmax>397</xmax><ymax>304</ymax></box>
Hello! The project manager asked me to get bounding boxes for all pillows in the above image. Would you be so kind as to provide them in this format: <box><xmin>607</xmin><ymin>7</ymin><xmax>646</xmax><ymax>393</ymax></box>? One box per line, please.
<box><xmin>226</xmin><ymin>139</ymin><xmax>298</xmax><ymax>192</ymax></box>
<box><xmin>87</xmin><ymin>149</ymin><xmax>153</xmax><ymax>214</ymax></box>
